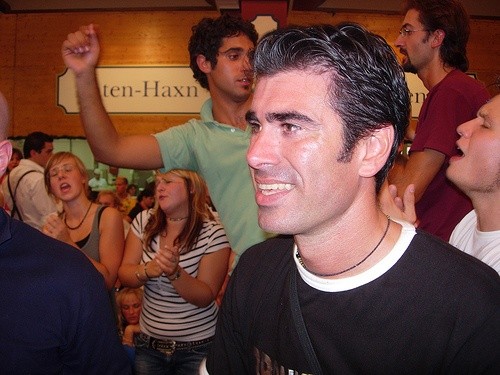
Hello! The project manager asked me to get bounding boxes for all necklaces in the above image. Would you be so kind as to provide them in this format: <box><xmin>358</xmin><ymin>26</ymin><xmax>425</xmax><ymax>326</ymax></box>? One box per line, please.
<box><xmin>296</xmin><ymin>219</ymin><xmax>390</xmax><ymax>277</ymax></box>
<box><xmin>64</xmin><ymin>201</ymin><xmax>93</xmax><ymax>230</ymax></box>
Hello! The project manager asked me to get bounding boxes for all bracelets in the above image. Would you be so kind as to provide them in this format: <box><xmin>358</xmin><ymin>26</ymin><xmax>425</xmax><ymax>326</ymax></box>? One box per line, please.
<box><xmin>136</xmin><ymin>273</ymin><xmax>143</xmax><ymax>283</ymax></box>
<box><xmin>145</xmin><ymin>261</ymin><xmax>151</xmax><ymax>278</ymax></box>
<box><xmin>169</xmin><ymin>272</ymin><xmax>180</xmax><ymax>281</ymax></box>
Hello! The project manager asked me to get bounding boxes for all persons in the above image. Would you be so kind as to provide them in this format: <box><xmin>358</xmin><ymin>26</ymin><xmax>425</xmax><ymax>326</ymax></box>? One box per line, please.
<box><xmin>128</xmin><ymin>182</ymin><xmax>156</xmax><ymax>222</ymax></box>
<box><xmin>0</xmin><ymin>131</ymin><xmax>57</xmax><ymax>233</ymax></box>
<box><xmin>378</xmin><ymin>93</ymin><xmax>500</xmax><ymax>274</ymax></box>
<box><xmin>0</xmin><ymin>93</ymin><xmax>122</xmax><ymax>375</ymax></box>
<box><xmin>206</xmin><ymin>22</ymin><xmax>500</xmax><ymax>375</ymax></box>
<box><xmin>115</xmin><ymin>175</ymin><xmax>137</xmax><ymax>213</ymax></box>
<box><xmin>6</xmin><ymin>148</ymin><xmax>23</xmax><ymax>172</ymax></box>
<box><xmin>62</xmin><ymin>14</ymin><xmax>277</xmax><ymax>255</ymax></box>
<box><xmin>116</xmin><ymin>170</ymin><xmax>231</xmax><ymax>375</ymax></box>
<box><xmin>96</xmin><ymin>190</ymin><xmax>123</xmax><ymax>210</ymax></box>
<box><xmin>88</xmin><ymin>169</ymin><xmax>106</xmax><ymax>187</ymax></box>
<box><xmin>41</xmin><ymin>151</ymin><xmax>125</xmax><ymax>289</ymax></box>
<box><xmin>387</xmin><ymin>0</ymin><xmax>493</xmax><ymax>242</ymax></box>
<box><xmin>116</xmin><ymin>287</ymin><xmax>144</xmax><ymax>358</ymax></box>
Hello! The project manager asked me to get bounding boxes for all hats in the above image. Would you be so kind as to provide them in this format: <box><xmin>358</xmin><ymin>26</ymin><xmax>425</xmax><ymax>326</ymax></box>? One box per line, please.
<box><xmin>95</xmin><ymin>168</ymin><xmax>101</xmax><ymax>175</ymax></box>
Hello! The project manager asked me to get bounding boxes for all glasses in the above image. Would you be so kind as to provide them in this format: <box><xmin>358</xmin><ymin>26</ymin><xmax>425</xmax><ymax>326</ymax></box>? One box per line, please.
<box><xmin>399</xmin><ymin>28</ymin><xmax>435</xmax><ymax>36</ymax></box>
<box><xmin>99</xmin><ymin>202</ymin><xmax>110</xmax><ymax>206</ymax></box>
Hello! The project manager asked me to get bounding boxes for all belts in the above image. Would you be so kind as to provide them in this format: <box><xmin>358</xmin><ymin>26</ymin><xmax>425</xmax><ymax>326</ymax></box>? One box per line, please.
<box><xmin>138</xmin><ymin>332</ymin><xmax>215</xmax><ymax>355</ymax></box>
